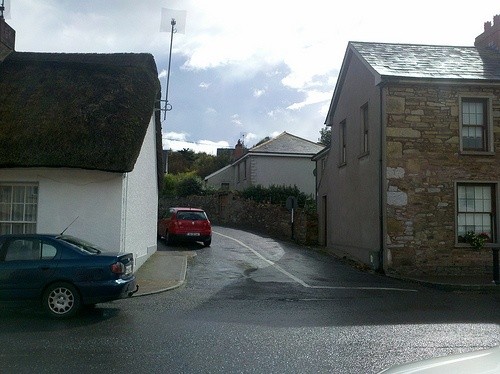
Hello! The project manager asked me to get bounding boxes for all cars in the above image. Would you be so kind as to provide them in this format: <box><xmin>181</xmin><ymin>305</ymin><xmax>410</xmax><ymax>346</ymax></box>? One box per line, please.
<box><xmin>158</xmin><ymin>206</ymin><xmax>212</xmax><ymax>247</ymax></box>
<box><xmin>0</xmin><ymin>216</ymin><xmax>136</xmax><ymax>320</ymax></box>
